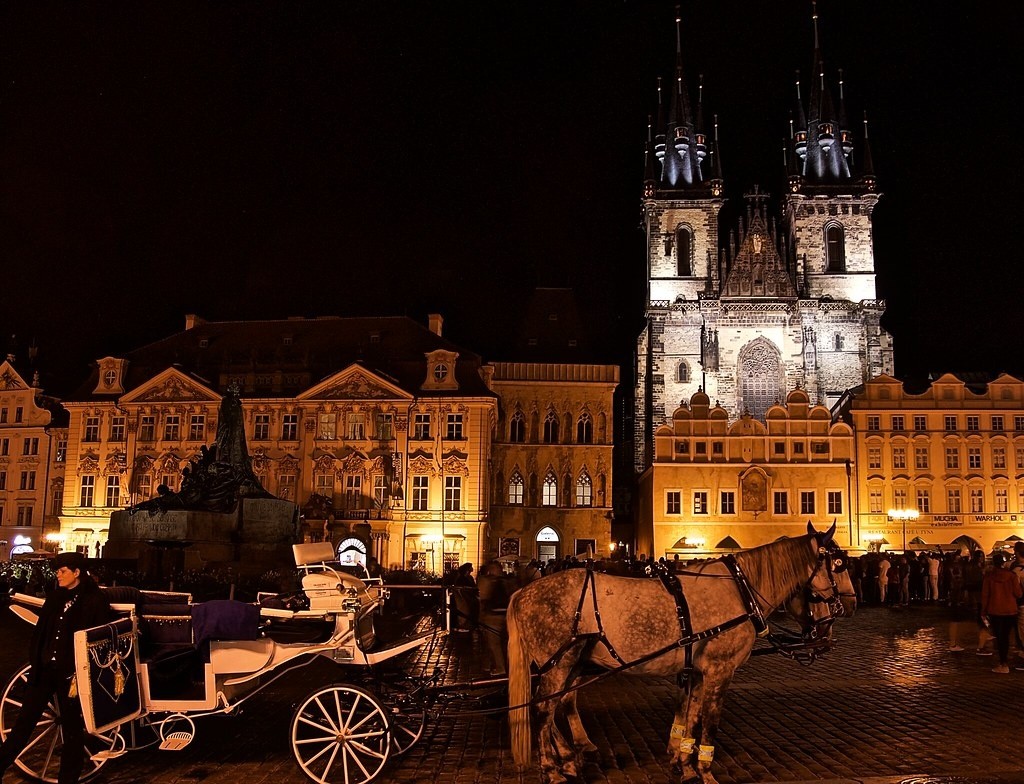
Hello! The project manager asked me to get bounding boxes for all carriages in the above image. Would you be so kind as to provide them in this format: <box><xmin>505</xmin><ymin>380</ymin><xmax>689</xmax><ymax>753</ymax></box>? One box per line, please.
<box><xmin>0</xmin><ymin>518</ymin><xmax>857</xmax><ymax>784</ymax></box>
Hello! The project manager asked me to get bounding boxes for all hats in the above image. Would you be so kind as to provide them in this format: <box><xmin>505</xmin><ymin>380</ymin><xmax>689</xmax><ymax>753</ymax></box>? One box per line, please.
<box><xmin>49</xmin><ymin>552</ymin><xmax>85</xmax><ymax>572</ymax></box>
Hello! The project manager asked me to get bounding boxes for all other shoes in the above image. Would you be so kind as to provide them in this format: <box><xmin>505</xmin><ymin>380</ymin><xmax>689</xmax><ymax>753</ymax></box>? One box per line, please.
<box><xmin>1015</xmin><ymin>663</ymin><xmax>1024</xmax><ymax>670</ymax></box>
<box><xmin>992</xmin><ymin>665</ymin><xmax>1010</xmax><ymax>674</ymax></box>
<box><xmin>481</xmin><ymin>664</ymin><xmax>491</xmax><ymax>671</ymax></box>
<box><xmin>454</xmin><ymin>628</ymin><xmax>469</xmax><ymax>632</ymax></box>
<box><xmin>949</xmin><ymin>645</ymin><xmax>965</xmax><ymax>651</ymax></box>
<box><xmin>490</xmin><ymin>669</ymin><xmax>507</xmax><ymax>675</ymax></box>
<box><xmin>976</xmin><ymin>648</ymin><xmax>993</xmax><ymax>655</ymax></box>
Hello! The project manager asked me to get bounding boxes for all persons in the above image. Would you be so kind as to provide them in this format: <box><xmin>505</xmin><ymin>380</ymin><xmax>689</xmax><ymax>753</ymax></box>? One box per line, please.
<box><xmin>845</xmin><ymin>551</ymin><xmax>966</xmax><ymax>604</ymax></box>
<box><xmin>0</xmin><ymin>561</ymin><xmax>55</xmax><ymax>625</ymax></box>
<box><xmin>0</xmin><ymin>551</ymin><xmax>113</xmax><ymax>781</ymax></box>
<box><xmin>942</xmin><ymin>542</ymin><xmax>1024</xmax><ymax>673</ymax></box>
<box><xmin>217</xmin><ymin>381</ymin><xmax>250</xmax><ymax>463</ymax></box>
<box><xmin>444</xmin><ymin>555</ymin><xmax>596</xmax><ymax>676</ymax></box>
<box><xmin>597</xmin><ymin>553</ymin><xmax>712</xmax><ymax>578</ymax></box>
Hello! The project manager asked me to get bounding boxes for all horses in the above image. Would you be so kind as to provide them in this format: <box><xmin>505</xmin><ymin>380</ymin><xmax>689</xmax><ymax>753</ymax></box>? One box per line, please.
<box><xmin>505</xmin><ymin>516</ymin><xmax>857</xmax><ymax>784</ymax></box>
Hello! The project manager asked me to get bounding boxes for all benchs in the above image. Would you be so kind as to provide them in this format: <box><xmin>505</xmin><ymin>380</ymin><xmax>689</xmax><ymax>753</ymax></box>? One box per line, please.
<box><xmin>103</xmin><ymin>584</ymin><xmax>194</xmax><ymax>644</ymax></box>
<box><xmin>292</xmin><ymin>543</ymin><xmax>379</xmax><ymax>609</ymax></box>
